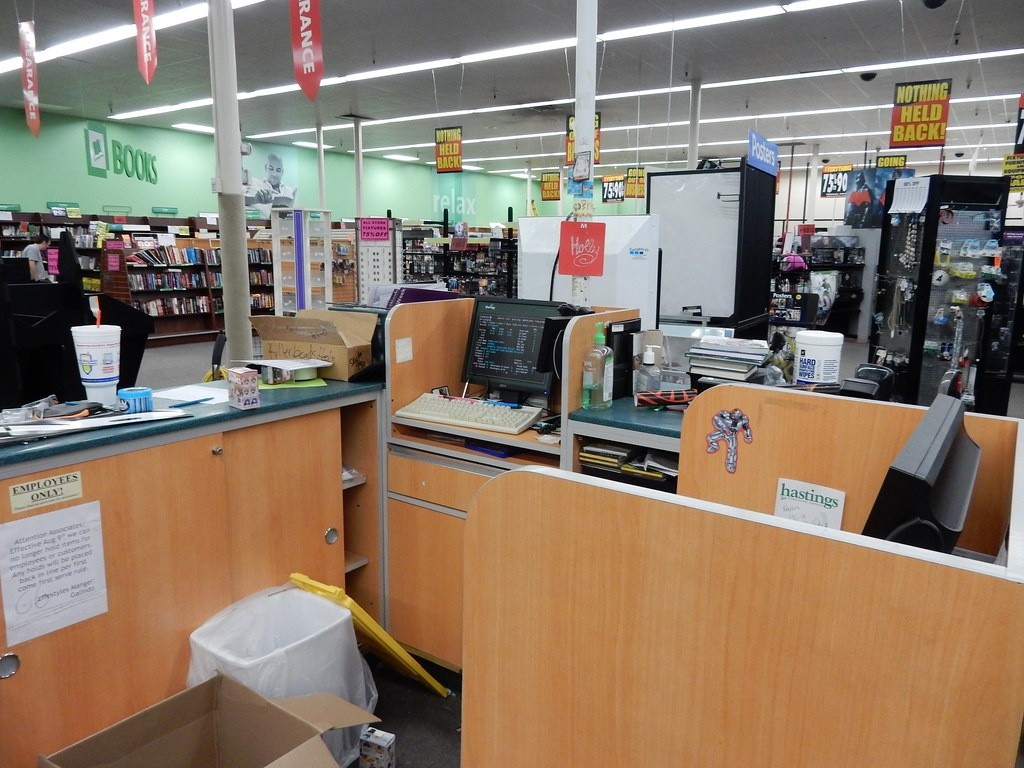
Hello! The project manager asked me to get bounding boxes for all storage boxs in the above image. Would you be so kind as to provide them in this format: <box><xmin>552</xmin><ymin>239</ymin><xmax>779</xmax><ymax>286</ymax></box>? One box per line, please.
<box><xmin>226</xmin><ymin>366</ymin><xmax>260</xmax><ymax>411</ymax></box>
<box><xmin>247</xmin><ymin>307</ymin><xmax>379</xmax><ymax>381</ymax></box>
<box><xmin>37</xmin><ymin>668</ymin><xmax>383</xmax><ymax>768</ymax></box>
<box><xmin>359</xmin><ymin>726</ymin><xmax>396</xmax><ymax>768</ymax></box>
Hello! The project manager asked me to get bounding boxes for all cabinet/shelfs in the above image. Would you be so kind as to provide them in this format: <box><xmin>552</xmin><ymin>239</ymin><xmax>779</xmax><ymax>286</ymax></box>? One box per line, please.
<box><xmin>386</xmin><ymin>443</ymin><xmax>508</xmax><ymax>677</ymax></box>
<box><xmin>0</xmin><ymin>207</ymin><xmax>519</xmax><ymax>347</ymax></box>
<box><xmin>0</xmin><ymin>378</ymin><xmax>386</xmax><ymax>768</ymax></box>
<box><xmin>775</xmin><ymin>244</ymin><xmax>865</xmax><ymax>339</ymax></box>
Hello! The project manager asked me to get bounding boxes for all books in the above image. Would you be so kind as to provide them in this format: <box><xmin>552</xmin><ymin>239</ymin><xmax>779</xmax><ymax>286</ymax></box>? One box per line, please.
<box><xmin>251</xmin><ymin>291</ymin><xmax>274</xmax><ymax>309</ymax></box>
<box><xmin>686</xmin><ymin>334</ymin><xmax>774</xmax><ymax>381</ymax></box>
<box><xmin>69</xmin><ymin>226</ymin><xmax>99</xmax><ymax>248</ymax></box>
<box><xmin>128</xmin><ymin>269</ymin><xmax>223</xmax><ymax>289</ymax></box>
<box><xmin>247</xmin><ymin>248</ymin><xmax>272</xmax><ymax>263</ymax></box>
<box><xmin>82</xmin><ymin>276</ymin><xmax>101</xmax><ymax>291</ymax></box>
<box><xmin>126</xmin><ymin>246</ymin><xmax>222</xmax><ymax>264</ymax></box>
<box><xmin>77</xmin><ymin>255</ymin><xmax>96</xmax><ymax>269</ymax></box>
<box><xmin>131</xmin><ymin>294</ymin><xmax>224</xmax><ymax>315</ymax></box>
<box><xmin>249</xmin><ymin>267</ymin><xmax>274</xmax><ymax>286</ymax></box>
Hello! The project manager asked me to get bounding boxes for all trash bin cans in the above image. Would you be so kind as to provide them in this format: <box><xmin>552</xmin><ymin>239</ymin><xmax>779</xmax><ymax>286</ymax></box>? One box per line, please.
<box><xmin>188</xmin><ymin>588</ymin><xmax>370</xmax><ymax>764</ymax></box>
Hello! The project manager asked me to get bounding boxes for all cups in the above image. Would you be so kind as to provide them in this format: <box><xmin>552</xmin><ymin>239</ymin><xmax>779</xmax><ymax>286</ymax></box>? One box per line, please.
<box><xmin>71</xmin><ymin>324</ymin><xmax>121</xmax><ymax>404</ymax></box>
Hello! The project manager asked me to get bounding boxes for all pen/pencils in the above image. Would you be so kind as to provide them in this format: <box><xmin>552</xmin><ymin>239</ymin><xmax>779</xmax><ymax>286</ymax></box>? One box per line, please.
<box><xmin>168</xmin><ymin>397</ymin><xmax>215</xmax><ymax>409</ymax></box>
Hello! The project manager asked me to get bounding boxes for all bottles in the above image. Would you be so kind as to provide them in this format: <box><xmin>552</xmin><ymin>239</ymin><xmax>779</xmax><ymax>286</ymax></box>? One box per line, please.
<box><xmin>637</xmin><ymin>344</ymin><xmax>661</xmax><ymax>390</ymax></box>
<box><xmin>583</xmin><ymin>322</ymin><xmax>614</xmax><ymax>410</ymax></box>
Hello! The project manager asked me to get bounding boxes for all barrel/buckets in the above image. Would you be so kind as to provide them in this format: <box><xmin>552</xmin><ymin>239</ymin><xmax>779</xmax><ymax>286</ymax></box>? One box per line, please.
<box><xmin>793</xmin><ymin>331</ymin><xmax>844</xmax><ymax>386</ymax></box>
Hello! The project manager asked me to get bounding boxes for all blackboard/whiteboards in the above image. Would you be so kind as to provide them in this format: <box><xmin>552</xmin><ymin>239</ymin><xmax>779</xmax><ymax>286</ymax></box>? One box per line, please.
<box><xmin>645</xmin><ymin>166</ymin><xmax>745</xmax><ymax>323</ymax></box>
<box><xmin>517</xmin><ymin>214</ymin><xmax>663</xmax><ymax>330</ymax></box>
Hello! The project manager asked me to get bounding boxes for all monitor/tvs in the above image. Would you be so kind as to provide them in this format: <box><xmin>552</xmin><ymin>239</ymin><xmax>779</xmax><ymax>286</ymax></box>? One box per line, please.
<box><xmin>461</xmin><ymin>295</ymin><xmax>567</xmax><ymax>404</ymax></box>
<box><xmin>861</xmin><ymin>393</ymin><xmax>982</xmax><ymax>553</ymax></box>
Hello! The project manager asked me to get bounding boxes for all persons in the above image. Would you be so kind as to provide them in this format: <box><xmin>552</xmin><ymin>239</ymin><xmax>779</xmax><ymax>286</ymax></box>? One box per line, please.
<box><xmin>21</xmin><ymin>235</ymin><xmax>50</xmax><ymax>282</ymax></box>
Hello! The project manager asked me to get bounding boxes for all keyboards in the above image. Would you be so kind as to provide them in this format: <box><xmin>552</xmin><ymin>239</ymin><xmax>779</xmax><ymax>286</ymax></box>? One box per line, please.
<box><xmin>395</xmin><ymin>393</ymin><xmax>543</xmax><ymax>435</ymax></box>
